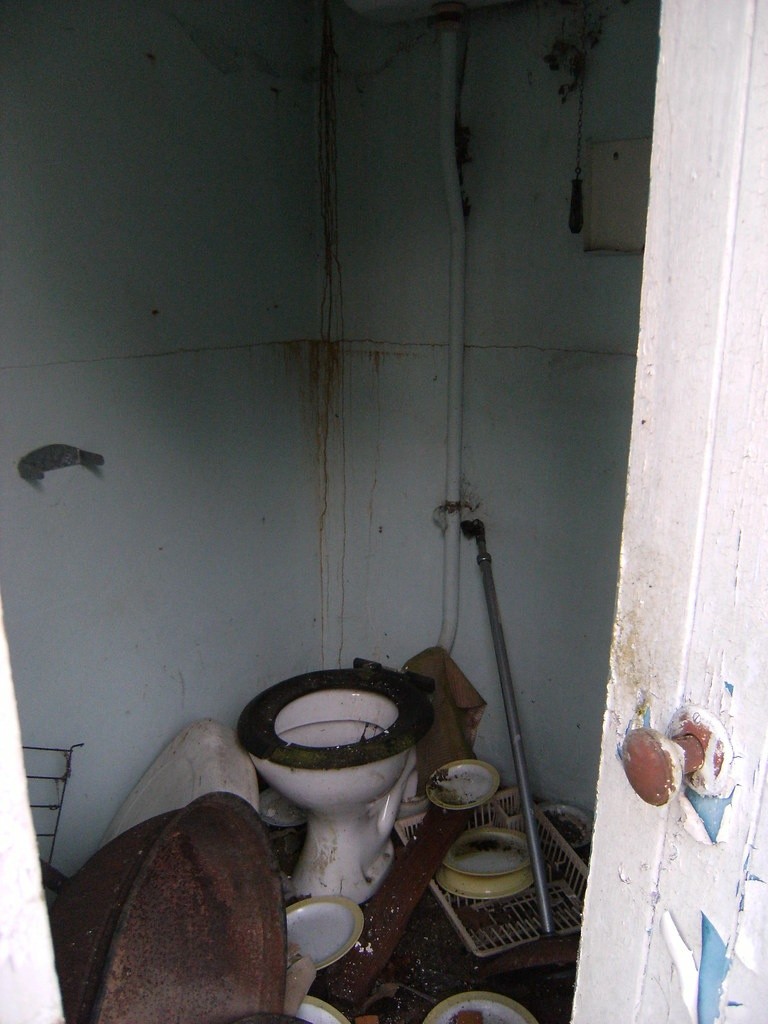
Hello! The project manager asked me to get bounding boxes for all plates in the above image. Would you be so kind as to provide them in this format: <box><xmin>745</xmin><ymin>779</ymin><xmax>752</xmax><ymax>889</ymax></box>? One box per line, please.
<box><xmin>436</xmin><ymin>867</ymin><xmax>534</xmax><ymax>900</ymax></box>
<box><xmin>420</xmin><ymin>990</ymin><xmax>539</xmax><ymax>1024</ymax></box>
<box><xmin>424</xmin><ymin>757</ymin><xmax>502</xmax><ymax>812</ymax></box>
<box><xmin>441</xmin><ymin>826</ymin><xmax>535</xmax><ymax>878</ymax></box>
<box><xmin>279</xmin><ymin>896</ymin><xmax>366</xmax><ymax>972</ymax></box>
<box><xmin>295</xmin><ymin>995</ymin><xmax>352</xmax><ymax>1024</ymax></box>
<box><xmin>532</xmin><ymin>803</ymin><xmax>595</xmax><ymax>849</ymax></box>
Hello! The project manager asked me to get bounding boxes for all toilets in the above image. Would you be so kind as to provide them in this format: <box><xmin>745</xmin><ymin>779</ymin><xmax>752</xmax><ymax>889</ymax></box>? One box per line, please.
<box><xmin>239</xmin><ymin>652</ymin><xmax>430</xmax><ymax>903</ymax></box>
